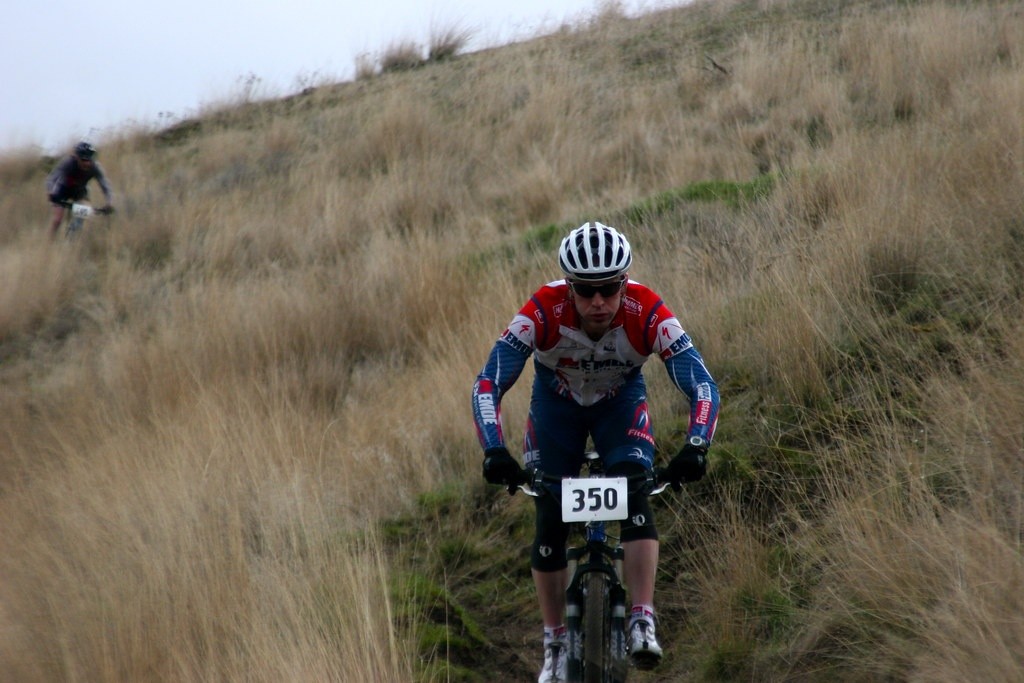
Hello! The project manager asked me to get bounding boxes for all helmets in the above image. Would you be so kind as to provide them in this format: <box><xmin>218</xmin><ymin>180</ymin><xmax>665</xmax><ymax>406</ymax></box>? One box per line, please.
<box><xmin>558</xmin><ymin>221</ymin><xmax>632</xmax><ymax>284</ymax></box>
<box><xmin>75</xmin><ymin>142</ymin><xmax>96</xmax><ymax>156</ymax></box>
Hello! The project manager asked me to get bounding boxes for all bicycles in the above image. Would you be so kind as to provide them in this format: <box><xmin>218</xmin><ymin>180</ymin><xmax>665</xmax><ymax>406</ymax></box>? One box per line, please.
<box><xmin>499</xmin><ymin>449</ymin><xmax>685</xmax><ymax>683</ymax></box>
<box><xmin>47</xmin><ymin>191</ymin><xmax>117</xmax><ymax>243</ymax></box>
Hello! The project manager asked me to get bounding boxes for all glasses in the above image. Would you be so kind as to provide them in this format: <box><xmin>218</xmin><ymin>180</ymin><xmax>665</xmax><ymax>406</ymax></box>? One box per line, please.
<box><xmin>567</xmin><ymin>274</ymin><xmax>625</xmax><ymax>299</ymax></box>
<box><xmin>79</xmin><ymin>157</ymin><xmax>92</xmax><ymax>162</ymax></box>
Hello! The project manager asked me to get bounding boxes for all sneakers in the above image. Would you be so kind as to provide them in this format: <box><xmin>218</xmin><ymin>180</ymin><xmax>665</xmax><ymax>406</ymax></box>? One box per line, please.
<box><xmin>538</xmin><ymin>630</ymin><xmax>569</xmax><ymax>683</ymax></box>
<box><xmin>625</xmin><ymin>616</ymin><xmax>662</xmax><ymax>671</ymax></box>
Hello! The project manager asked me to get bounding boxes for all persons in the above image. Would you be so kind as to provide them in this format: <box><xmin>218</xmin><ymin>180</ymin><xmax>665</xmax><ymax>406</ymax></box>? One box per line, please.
<box><xmin>47</xmin><ymin>141</ymin><xmax>110</xmax><ymax>241</ymax></box>
<box><xmin>471</xmin><ymin>221</ymin><xmax>721</xmax><ymax>683</ymax></box>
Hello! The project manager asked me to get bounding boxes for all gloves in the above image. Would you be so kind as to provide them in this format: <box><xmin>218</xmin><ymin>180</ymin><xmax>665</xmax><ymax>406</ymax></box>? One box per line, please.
<box><xmin>483</xmin><ymin>449</ymin><xmax>522</xmax><ymax>495</ymax></box>
<box><xmin>102</xmin><ymin>205</ymin><xmax>112</xmax><ymax>215</ymax></box>
<box><xmin>655</xmin><ymin>444</ymin><xmax>706</xmax><ymax>495</ymax></box>
<box><xmin>49</xmin><ymin>194</ymin><xmax>61</xmax><ymax>204</ymax></box>
